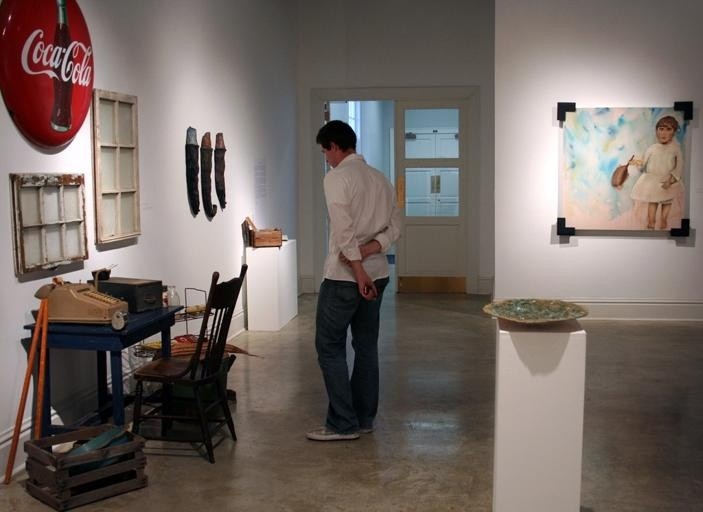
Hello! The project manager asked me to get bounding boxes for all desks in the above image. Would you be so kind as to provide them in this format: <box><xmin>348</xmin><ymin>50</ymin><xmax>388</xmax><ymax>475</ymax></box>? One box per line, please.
<box><xmin>23</xmin><ymin>306</ymin><xmax>184</xmax><ymax>439</ymax></box>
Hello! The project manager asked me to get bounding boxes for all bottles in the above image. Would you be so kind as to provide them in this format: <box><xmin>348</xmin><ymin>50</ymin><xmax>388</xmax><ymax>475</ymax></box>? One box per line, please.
<box><xmin>162</xmin><ymin>285</ymin><xmax>168</xmax><ymax>308</ymax></box>
<box><xmin>168</xmin><ymin>286</ymin><xmax>181</xmax><ymax>320</ymax></box>
<box><xmin>50</xmin><ymin>0</ymin><xmax>74</xmax><ymax>133</ymax></box>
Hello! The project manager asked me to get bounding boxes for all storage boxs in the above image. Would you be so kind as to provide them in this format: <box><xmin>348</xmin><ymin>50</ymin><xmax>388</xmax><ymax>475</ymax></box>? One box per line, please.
<box><xmin>23</xmin><ymin>423</ymin><xmax>148</xmax><ymax>512</ymax></box>
<box><xmin>249</xmin><ymin>229</ymin><xmax>282</xmax><ymax>247</ymax></box>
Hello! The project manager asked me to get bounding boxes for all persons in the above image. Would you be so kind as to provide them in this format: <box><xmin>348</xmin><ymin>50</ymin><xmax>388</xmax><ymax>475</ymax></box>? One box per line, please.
<box><xmin>627</xmin><ymin>113</ymin><xmax>682</xmax><ymax>229</ymax></box>
<box><xmin>302</xmin><ymin>120</ymin><xmax>400</xmax><ymax>443</ymax></box>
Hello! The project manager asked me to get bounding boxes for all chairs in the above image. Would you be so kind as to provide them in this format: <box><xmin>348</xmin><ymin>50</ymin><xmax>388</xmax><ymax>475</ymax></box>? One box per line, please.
<box><xmin>130</xmin><ymin>265</ymin><xmax>247</xmax><ymax>464</ymax></box>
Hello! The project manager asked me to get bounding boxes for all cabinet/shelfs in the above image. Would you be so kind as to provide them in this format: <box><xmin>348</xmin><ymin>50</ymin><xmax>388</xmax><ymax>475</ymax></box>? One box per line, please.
<box><xmin>246</xmin><ymin>238</ymin><xmax>297</xmax><ymax>333</ymax></box>
<box><xmin>493</xmin><ymin>318</ymin><xmax>587</xmax><ymax>512</ymax></box>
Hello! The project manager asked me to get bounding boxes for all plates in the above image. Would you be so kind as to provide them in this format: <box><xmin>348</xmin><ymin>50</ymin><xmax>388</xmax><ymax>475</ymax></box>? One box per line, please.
<box><xmin>479</xmin><ymin>296</ymin><xmax>590</xmax><ymax>325</ymax></box>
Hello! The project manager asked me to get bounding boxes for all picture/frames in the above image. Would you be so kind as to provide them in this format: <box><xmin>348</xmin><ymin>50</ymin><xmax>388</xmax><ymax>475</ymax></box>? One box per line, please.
<box><xmin>557</xmin><ymin>102</ymin><xmax>694</xmax><ymax>237</ymax></box>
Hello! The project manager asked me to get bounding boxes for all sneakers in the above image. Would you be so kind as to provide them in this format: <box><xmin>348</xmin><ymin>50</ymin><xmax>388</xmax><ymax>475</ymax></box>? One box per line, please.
<box><xmin>305</xmin><ymin>422</ymin><xmax>375</xmax><ymax>441</ymax></box>
